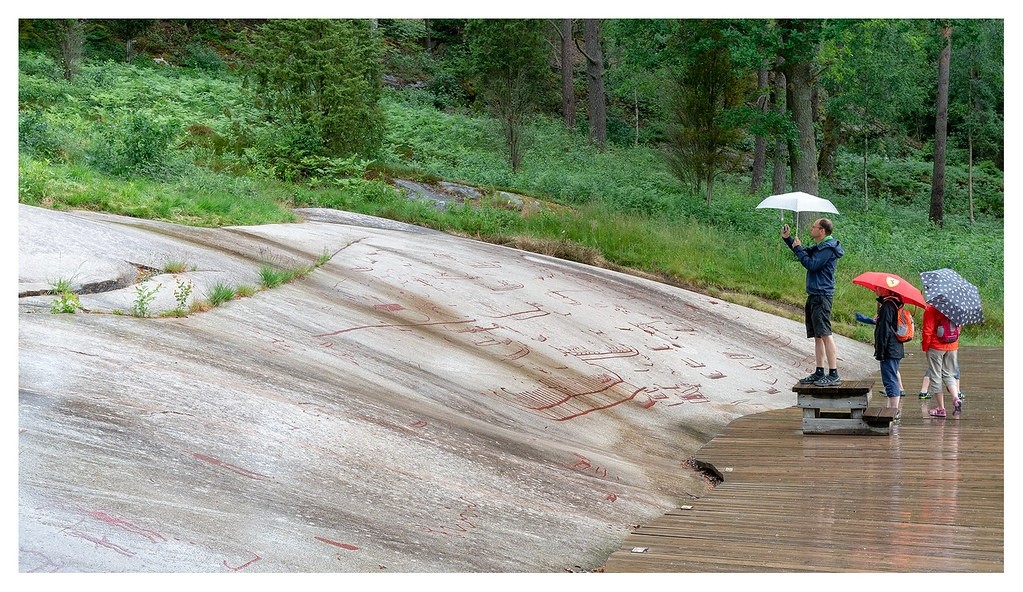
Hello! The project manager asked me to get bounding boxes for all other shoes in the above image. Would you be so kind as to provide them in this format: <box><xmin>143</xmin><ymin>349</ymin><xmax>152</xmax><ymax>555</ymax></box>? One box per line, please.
<box><xmin>880</xmin><ymin>388</ymin><xmax>906</xmax><ymax>396</ymax></box>
<box><xmin>894</xmin><ymin>413</ymin><xmax>901</xmax><ymax>422</ymax></box>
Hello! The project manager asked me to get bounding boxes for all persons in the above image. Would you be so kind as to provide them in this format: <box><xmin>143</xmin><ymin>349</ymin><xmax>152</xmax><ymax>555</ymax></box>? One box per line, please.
<box><xmin>781</xmin><ymin>218</ymin><xmax>844</xmax><ymax>386</ymax></box>
<box><xmin>918</xmin><ymin>361</ymin><xmax>966</xmax><ymax>399</ymax></box>
<box><xmin>922</xmin><ymin>304</ymin><xmax>962</xmax><ymax>417</ymax></box>
<box><xmin>873</xmin><ymin>286</ymin><xmax>905</xmax><ymax>422</ymax></box>
<box><xmin>879</xmin><ymin>370</ymin><xmax>906</xmax><ymax>396</ymax></box>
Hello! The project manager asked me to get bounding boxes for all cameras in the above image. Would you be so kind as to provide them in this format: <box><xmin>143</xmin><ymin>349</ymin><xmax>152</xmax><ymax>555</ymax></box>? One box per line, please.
<box><xmin>784</xmin><ymin>225</ymin><xmax>787</xmax><ymax>232</ymax></box>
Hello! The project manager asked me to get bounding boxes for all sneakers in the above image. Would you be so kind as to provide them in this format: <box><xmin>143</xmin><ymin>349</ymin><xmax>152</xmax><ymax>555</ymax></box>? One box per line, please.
<box><xmin>928</xmin><ymin>408</ymin><xmax>947</xmax><ymax>417</ymax></box>
<box><xmin>918</xmin><ymin>392</ymin><xmax>932</xmax><ymax>399</ymax></box>
<box><xmin>800</xmin><ymin>374</ymin><xmax>821</xmax><ymax>384</ymax></box>
<box><xmin>813</xmin><ymin>376</ymin><xmax>841</xmax><ymax>385</ymax></box>
<box><xmin>952</xmin><ymin>398</ymin><xmax>962</xmax><ymax>418</ymax></box>
<box><xmin>957</xmin><ymin>392</ymin><xmax>965</xmax><ymax>399</ymax></box>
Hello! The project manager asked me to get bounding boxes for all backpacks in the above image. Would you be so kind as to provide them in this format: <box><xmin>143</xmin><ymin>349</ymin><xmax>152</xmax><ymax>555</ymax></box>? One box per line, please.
<box><xmin>935</xmin><ymin>315</ymin><xmax>959</xmax><ymax>343</ymax></box>
<box><xmin>881</xmin><ymin>299</ymin><xmax>913</xmax><ymax>342</ymax></box>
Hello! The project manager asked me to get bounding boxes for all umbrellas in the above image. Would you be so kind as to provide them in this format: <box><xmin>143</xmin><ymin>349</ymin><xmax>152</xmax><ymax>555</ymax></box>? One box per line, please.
<box><xmin>755</xmin><ymin>191</ymin><xmax>839</xmax><ymax>236</ymax></box>
<box><xmin>920</xmin><ymin>268</ymin><xmax>984</xmax><ymax>327</ymax></box>
<box><xmin>852</xmin><ymin>271</ymin><xmax>928</xmax><ymax>316</ymax></box>
